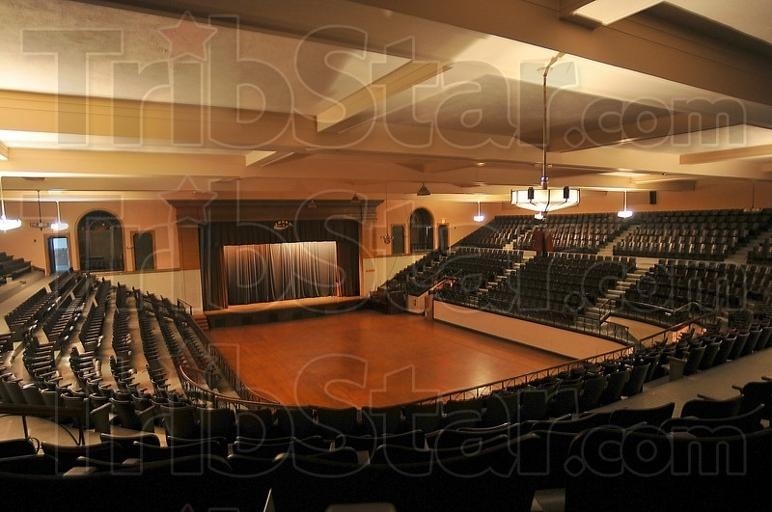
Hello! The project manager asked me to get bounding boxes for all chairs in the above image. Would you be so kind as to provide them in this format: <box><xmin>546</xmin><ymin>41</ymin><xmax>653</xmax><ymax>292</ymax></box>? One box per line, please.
<box><xmin>0</xmin><ymin>211</ymin><xmax>772</xmax><ymax>512</ymax></box>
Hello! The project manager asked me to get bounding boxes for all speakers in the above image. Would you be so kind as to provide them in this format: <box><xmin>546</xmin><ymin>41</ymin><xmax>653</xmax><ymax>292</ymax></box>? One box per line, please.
<box><xmin>649</xmin><ymin>190</ymin><xmax>657</xmax><ymax>204</ymax></box>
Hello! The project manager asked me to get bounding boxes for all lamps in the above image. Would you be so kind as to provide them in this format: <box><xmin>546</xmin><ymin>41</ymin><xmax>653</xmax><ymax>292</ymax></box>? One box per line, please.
<box><xmin>51</xmin><ymin>201</ymin><xmax>69</xmax><ymax>231</ymax></box>
<box><xmin>473</xmin><ymin>202</ymin><xmax>484</xmax><ymax>222</ymax></box>
<box><xmin>417</xmin><ymin>181</ymin><xmax>431</xmax><ymax>196</ymax></box>
<box><xmin>618</xmin><ymin>192</ymin><xmax>633</xmax><ymax>219</ymax></box>
<box><xmin>510</xmin><ymin>50</ymin><xmax>581</xmax><ymax>220</ymax></box>
<box><xmin>0</xmin><ymin>199</ymin><xmax>22</xmax><ymax>230</ymax></box>
